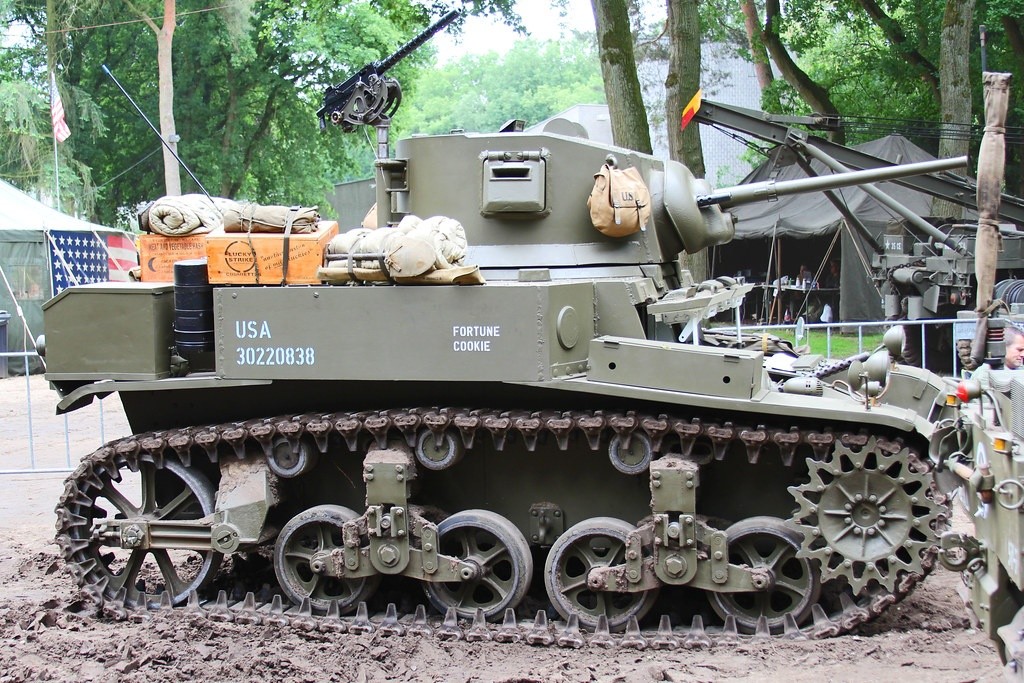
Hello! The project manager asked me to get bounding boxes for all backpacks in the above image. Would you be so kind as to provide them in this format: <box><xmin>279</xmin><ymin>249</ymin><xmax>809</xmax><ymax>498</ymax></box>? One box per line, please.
<box><xmin>588</xmin><ymin>164</ymin><xmax>652</xmax><ymax>236</ymax></box>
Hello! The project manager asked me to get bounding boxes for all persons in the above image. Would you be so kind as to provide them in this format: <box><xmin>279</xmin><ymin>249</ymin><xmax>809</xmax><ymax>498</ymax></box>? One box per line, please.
<box><xmin>783</xmin><ymin>259</ymin><xmax>841</xmax><ymax>324</ymax></box>
<box><xmin>957</xmin><ymin>328</ymin><xmax>1024</xmax><ymax>628</ymax></box>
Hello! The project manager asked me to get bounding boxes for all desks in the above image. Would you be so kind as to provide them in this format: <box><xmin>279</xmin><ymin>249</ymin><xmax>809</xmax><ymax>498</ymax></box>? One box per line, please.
<box><xmin>725</xmin><ymin>283</ymin><xmax>763</xmax><ymax>323</ymax></box>
<box><xmin>760</xmin><ymin>284</ymin><xmax>839</xmax><ymax>323</ymax></box>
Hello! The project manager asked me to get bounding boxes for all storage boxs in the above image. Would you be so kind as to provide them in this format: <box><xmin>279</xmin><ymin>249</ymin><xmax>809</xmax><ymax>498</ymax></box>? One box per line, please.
<box><xmin>138</xmin><ymin>233</ymin><xmax>208</xmax><ymax>283</ymax></box>
<box><xmin>206</xmin><ymin>219</ymin><xmax>339</xmax><ymax>286</ymax></box>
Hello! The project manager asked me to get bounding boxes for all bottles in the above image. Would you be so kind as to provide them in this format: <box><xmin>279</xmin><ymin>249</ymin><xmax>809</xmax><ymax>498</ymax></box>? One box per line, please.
<box><xmin>813</xmin><ymin>280</ymin><xmax>816</xmax><ymax>289</ymax></box>
<box><xmin>789</xmin><ymin>278</ymin><xmax>792</xmax><ymax>287</ymax></box>
<box><xmin>796</xmin><ymin>275</ymin><xmax>800</xmax><ymax>288</ymax></box>
<box><xmin>803</xmin><ymin>278</ymin><xmax>807</xmax><ymax>289</ymax></box>
<box><xmin>784</xmin><ymin>277</ymin><xmax>788</xmax><ymax>287</ymax></box>
<box><xmin>784</xmin><ymin>308</ymin><xmax>790</xmax><ymax>324</ymax></box>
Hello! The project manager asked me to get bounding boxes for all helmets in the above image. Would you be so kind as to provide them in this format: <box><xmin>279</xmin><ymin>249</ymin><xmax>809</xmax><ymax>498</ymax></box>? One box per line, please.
<box><xmin>389</xmin><ymin>238</ymin><xmax>436</xmax><ymax>277</ymax></box>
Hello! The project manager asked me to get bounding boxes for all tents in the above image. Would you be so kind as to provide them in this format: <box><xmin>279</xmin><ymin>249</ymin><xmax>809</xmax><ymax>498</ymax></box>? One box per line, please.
<box><xmin>0</xmin><ymin>180</ymin><xmax>136</xmax><ymax>377</ymax></box>
<box><xmin>707</xmin><ymin>131</ymin><xmax>1023</xmax><ymax>333</ymax></box>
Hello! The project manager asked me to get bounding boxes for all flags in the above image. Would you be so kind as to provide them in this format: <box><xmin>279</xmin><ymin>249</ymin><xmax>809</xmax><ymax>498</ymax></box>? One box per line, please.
<box><xmin>51</xmin><ymin>75</ymin><xmax>71</xmax><ymax>141</ymax></box>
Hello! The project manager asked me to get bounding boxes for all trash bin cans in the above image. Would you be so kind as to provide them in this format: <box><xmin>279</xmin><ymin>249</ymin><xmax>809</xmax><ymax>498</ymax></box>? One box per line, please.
<box><xmin>0</xmin><ymin>309</ymin><xmax>12</xmax><ymax>380</ymax></box>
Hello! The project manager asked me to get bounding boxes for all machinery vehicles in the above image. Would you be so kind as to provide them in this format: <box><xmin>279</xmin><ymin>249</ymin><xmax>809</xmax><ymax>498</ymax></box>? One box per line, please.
<box><xmin>34</xmin><ymin>13</ymin><xmax>971</xmax><ymax>641</ymax></box>
<box><xmin>676</xmin><ymin>89</ymin><xmax>1024</xmax><ymax>374</ymax></box>
